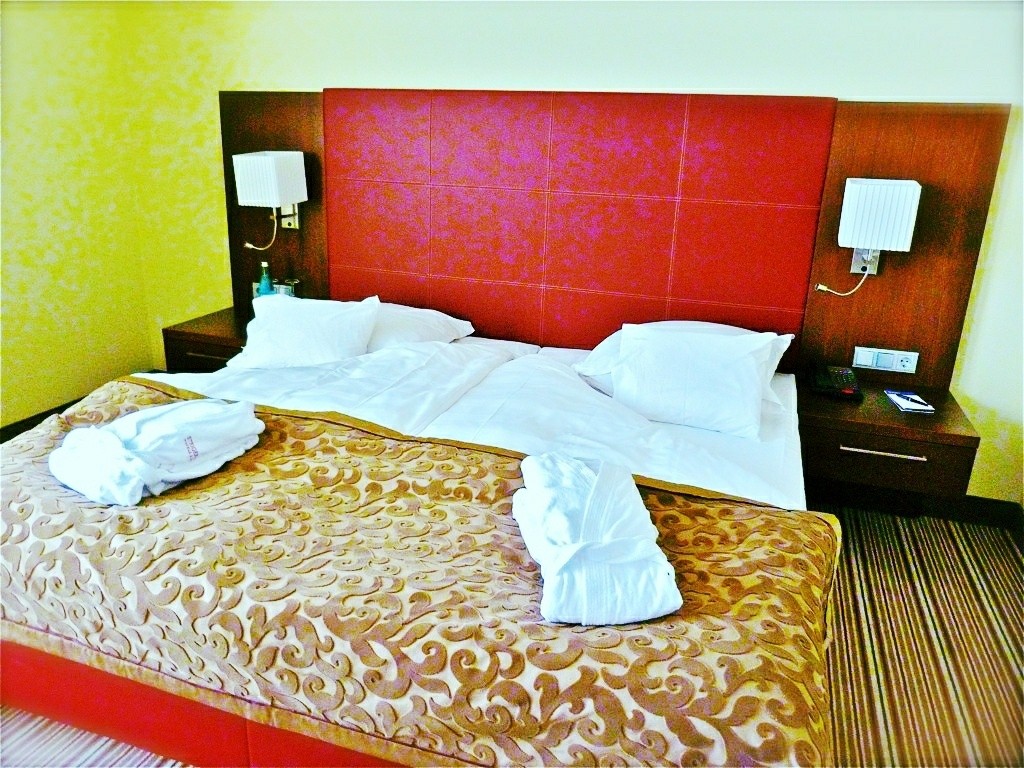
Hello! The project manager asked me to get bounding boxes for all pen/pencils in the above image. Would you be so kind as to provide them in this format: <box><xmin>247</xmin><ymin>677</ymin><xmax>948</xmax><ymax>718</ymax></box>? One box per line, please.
<box><xmin>896</xmin><ymin>393</ymin><xmax>928</xmax><ymax>406</ymax></box>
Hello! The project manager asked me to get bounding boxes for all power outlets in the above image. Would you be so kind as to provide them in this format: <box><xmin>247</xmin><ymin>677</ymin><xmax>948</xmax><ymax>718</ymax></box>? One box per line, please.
<box><xmin>252</xmin><ymin>283</ymin><xmax>262</xmax><ymax>298</ymax></box>
<box><xmin>894</xmin><ymin>351</ymin><xmax>920</xmax><ymax>373</ymax></box>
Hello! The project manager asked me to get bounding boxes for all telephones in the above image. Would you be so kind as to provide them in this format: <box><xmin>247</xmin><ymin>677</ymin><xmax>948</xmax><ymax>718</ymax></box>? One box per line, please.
<box><xmin>807</xmin><ymin>358</ymin><xmax>863</xmax><ymax>400</ymax></box>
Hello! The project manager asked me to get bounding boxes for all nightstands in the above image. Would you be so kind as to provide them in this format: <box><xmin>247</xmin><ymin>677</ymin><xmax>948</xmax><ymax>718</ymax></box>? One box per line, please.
<box><xmin>796</xmin><ymin>363</ymin><xmax>983</xmax><ymax>520</ymax></box>
<box><xmin>161</xmin><ymin>303</ymin><xmax>257</xmax><ymax>374</ymax></box>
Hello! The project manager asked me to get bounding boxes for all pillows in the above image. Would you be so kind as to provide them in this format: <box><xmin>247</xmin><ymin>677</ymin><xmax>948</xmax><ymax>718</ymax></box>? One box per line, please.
<box><xmin>611</xmin><ymin>321</ymin><xmax>778</xmax><ymax>443</ymax></box>
<box><xmin>570</xmin><ymin>320</ymin><xmax>794</xmax><ymax>410</ymax></box>
<box><xmin>227</xmin><ymin>293</ymin><xmax>381</xmax><ymax>368</ymax></box>
<box><xmin>363</xmin><ymin>300</ymin><xmax>476</xmax><ymax>355</ymax></box>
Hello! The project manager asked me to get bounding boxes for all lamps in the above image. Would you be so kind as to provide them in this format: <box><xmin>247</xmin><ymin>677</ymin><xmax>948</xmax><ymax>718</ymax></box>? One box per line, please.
<box><xmin>231</xmin><ymin>149</ymin><xmax>310</xmax><ymax>251</ymax></box>
<box><xmin>815</xmin><ymin>177</ymin><xmax>923</xmax><ymax>297</ymax></box>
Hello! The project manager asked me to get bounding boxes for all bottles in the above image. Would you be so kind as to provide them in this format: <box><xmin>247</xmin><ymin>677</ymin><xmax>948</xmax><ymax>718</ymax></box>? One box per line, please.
<box><xmin>259</xmin><ymin>262</ymin><xmax>271</xmax><ymax>296</ymax></box>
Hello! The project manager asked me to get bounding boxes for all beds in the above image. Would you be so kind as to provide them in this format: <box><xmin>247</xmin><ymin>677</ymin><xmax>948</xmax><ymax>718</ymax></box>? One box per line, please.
<box><xmin>0</xmin><ymin>85</ymin><xmax>843</xmax><ymax>768</ymax></box>
